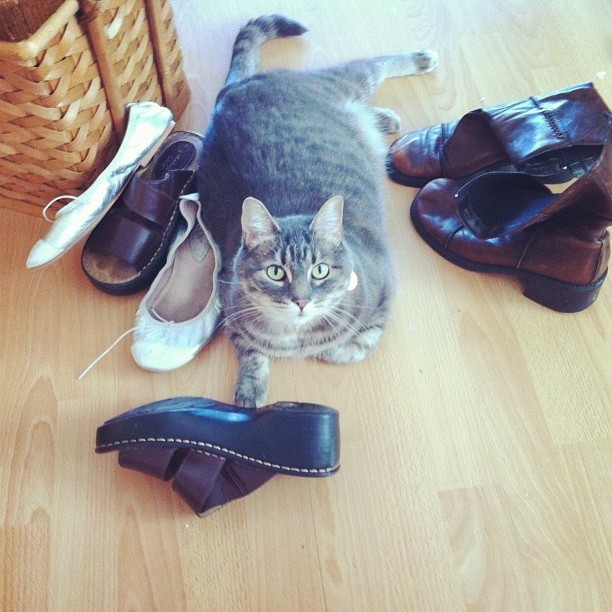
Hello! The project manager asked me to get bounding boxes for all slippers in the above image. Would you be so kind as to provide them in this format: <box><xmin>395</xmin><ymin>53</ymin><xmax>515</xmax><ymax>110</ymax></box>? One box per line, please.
<box><xmin>95</xmin><ymin>398</ymin><xmax>339</xmax><ymax>518</ymax></box>
<box><xmin>82</xmin><ymin>130</ymin><xmax>205</xmax><ymax>296</ymax></box>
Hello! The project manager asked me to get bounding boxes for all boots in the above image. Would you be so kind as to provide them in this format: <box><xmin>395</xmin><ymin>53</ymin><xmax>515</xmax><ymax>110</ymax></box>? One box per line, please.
<box><xmin>386</xmin><ymin>83</ymin><xmax>612</xmax><ymax>188</ymax></box>
<box><xmin>409</xmin><ymin>145</ymin><xmax>612</xmax><ymax>313</ymax></box>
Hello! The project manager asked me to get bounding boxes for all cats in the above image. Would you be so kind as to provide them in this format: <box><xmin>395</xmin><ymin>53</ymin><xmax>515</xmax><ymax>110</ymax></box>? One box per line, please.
<box><xmin>196</xmin><ymin>12</ymin><xmax>437</xmax><ymax>410</ymax></box>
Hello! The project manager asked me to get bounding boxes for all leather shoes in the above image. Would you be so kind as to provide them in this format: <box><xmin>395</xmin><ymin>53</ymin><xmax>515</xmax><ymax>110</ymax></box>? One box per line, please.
<box><xmin>129</xmin><ymin>193</ymin><xmax>224</xmax><ymax>372</ymax></box>
<box><xmin>25</xmin><ymin>101</ymin><xmax>175</xmax><ymax>271</ymax></box>
